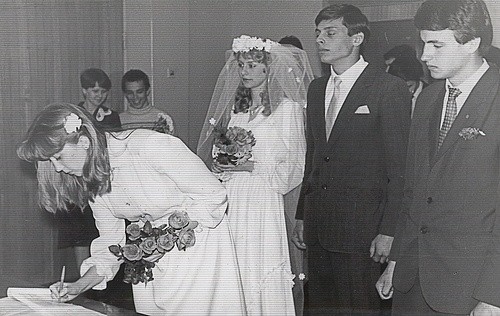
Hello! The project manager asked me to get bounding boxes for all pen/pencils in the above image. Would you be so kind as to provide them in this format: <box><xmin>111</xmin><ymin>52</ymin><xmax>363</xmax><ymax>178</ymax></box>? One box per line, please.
<box><xmin>56</xmin><ymin>265</ymin><xmax>68</xmax><ymax>304</ymax></box>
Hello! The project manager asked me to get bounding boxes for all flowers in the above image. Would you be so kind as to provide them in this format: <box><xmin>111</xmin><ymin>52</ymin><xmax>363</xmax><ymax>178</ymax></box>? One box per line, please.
<box><xmin>106</xmin><ymin>210</ymin><xmax>198</xmax><ymax>285</ymax></box>
<box><xmin>458</xmin><ymin>127</ymin><xmax>487</xmax><ymax>141</ymax></box>
<box><xmin>209</xmin><ymin>124</ymin><xmax>256</xmax><ymax>180</ymax></box>
<box><xmin>152</xmin><ymin>112</ymin><xmax>175</xmax><ymax>136</ymax></box>
<box><xmin>232</xmin><ymin>34</ymin><xmax>279</xmax><ymax>53</ymax></box>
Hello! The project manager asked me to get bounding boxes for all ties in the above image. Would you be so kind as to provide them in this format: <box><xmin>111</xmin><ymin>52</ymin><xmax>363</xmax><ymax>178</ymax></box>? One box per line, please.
<box><xmin>437</xmin><ymin>85</ymin><xmax>462</xmax><ymax>152</ymax></box>
<box><xmin>326</xmin><ymin>77</ymin><xmax>342</xmax><ymax>142</ymax></box>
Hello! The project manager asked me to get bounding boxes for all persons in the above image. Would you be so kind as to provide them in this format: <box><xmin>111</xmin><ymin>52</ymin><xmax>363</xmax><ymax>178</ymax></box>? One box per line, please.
<box><xmin>58</xmin><ymin>69</ymin><xmax>122</xmax><ymax>269</ymax></box>
<box><xmin>290</xmin><ymin>5</ymin><xmax>412</xmax><ymax>316</ymax></box>
<box><xmin>119</xmin><ymin>69</ymin><xmax>174</xmax><ymax>136</ymax></box>
<box><xmin>384</xmin><ymin>45</ymin><xmax>431</xmax><ymax>113</ymax></box>
<box><xmin>375</xmin><ymin>0</ymin><xmax>500</xmax><ymax>316</ymax></box>
<box><xmin>197</xmin><ymin>34</ymin><xmax>315</xmax><ymax>316</ymax></box>
<box><xmin>17</xmin><ymin>103</ymin><xmax>247</xmax><ymax>316</ymax></box>
